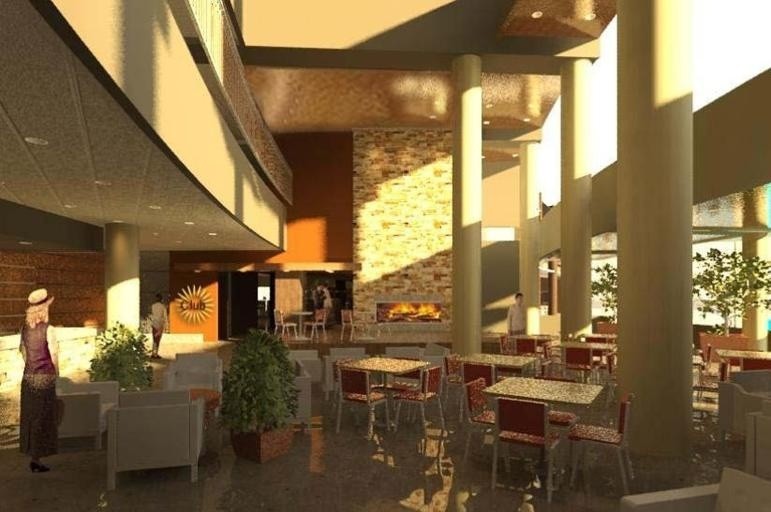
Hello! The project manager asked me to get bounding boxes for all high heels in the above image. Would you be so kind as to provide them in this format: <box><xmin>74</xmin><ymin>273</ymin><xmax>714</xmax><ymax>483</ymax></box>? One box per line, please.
<box><xmin>30</xmin><ymin>461</ymin><xmax>49</xmax><ymax>473</ymax></box>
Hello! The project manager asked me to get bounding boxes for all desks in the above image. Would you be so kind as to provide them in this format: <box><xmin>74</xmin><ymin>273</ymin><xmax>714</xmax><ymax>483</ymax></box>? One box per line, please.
<box><xmin>458</xmin><ymin>352</ymin><xmax>537</xmax><ymax>401</ymax></box>
<box><xmin>480</xmin><ymin>376</ymin><xmax>605</xmax><ymax>489</ymax></box>
<box><xmin>337</xmin><ymin>356</ymin><xmax>430</xmax><ymax>432</ymax></box>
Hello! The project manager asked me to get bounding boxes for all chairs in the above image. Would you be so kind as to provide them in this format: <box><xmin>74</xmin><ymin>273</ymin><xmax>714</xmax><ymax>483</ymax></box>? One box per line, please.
<box><xmin>320</xmin><ymin>355</ymin><xmax>338</xmax><ymax>401</ymax></box>
<box><xmin>444</xmin><ymin>354</ymin><xmax>460</xmax><ymax>404</ymax></box>
<box><xmin>488</xmin><ymin>397</ymin><xmax>554</xmax><ymax>505</ymax></box>
<box><xmin>393</xmin><ymin>356</ymin><xmax>422</xmax><ymax>411</ymax></box>
<box><xmin>332</xmin><ymin>359</ymin><xmax>352</xmax><ymax>408</ymax></box>
<box><xmin>49</xmin><ymin>373</ymin><xmax>119</xmax><ymax>450</ymax></box>
<box><xmin>546</xmin><ymin>407</ymin><xmax>575</xmax><ymax>425</ymax></box>
<box><xmin>337</xmin><ymin>367</ymin><xmax>390</xmax><ymax>439</ymax></box>
<box><xmin>104</xmin><ymin>386</ymin><xmax>205</xmax><ymax>491</ymax></box>
<box><xmin>270</xmin><ymin>307</ymin><xmax>393</xmax><ymax>342</ymax></box>
<box><xmin>459</xmin><ymin>362</ymin><xmax>497</xmax><ymax>423</ymax></box>
<box><xmin>365</xmin><ymin>343</ymin><xmax>387</xmax><ymax>357</ymax></box>
<box><xmin>621</xmin><ymin>336</ymin><xmax>771</xmax><ymax>511</ymax></box>
<box><xmin>392</xmin><ymin>366</ymin><xmax>444</xmax><ymax>429</ymax></box>
<box><xmin>481</xmin><ymin>336</ymin><xmax>621</xmax><ymax>382</ymax></box>
<box><xmin>566</xmin><ymin>390</ymin><xmax>633</xmax><ymax>494</ymax></box>
<box><xmin>463</xmin><ymin>377</ymin><xmax>504</xmax><ymax>466</ymax></box>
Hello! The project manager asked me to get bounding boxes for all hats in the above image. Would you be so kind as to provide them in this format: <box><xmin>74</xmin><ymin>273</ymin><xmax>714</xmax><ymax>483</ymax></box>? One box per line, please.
<box><xmin>26</xmin><ymin>288</ymin><xmax>55</xmax><ymax>312</ymax></box>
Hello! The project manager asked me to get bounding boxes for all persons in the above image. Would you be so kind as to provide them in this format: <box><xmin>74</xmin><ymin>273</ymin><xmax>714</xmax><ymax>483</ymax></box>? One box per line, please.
<box><xmin>310</xmin><ymin>281</ymin><xmax>352</xmax><ymax>329</ymax></box>
<box><xmin>506</xmin><ymin>291</ymin><xmax>528</xmax><ymax>336</ymax></box>
<box><xmin>149</xmin><ymin>292</ymin><xmax>169</xmax><ymax>361</ymax></box>
<box><xmin>17</xmin><ymin>287</ymin><xmax>61</xmax><ymax>475</ymax></box>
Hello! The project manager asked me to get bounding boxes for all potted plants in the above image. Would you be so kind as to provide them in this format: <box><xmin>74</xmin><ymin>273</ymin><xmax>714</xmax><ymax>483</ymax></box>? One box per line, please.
<box><xmin>589</xmin><ymin>264</ymin><xmax>619</xmax><ymax>337</ymax></box>
<box><xmin>693</xmin><ymin>248</ymin><xmax>771</xmax><ymax>364</ymax></box>
<box><xmin>218</xmin><ymin>325</ymin><xmax>301</xmax><ymax>463</ymax></box>
